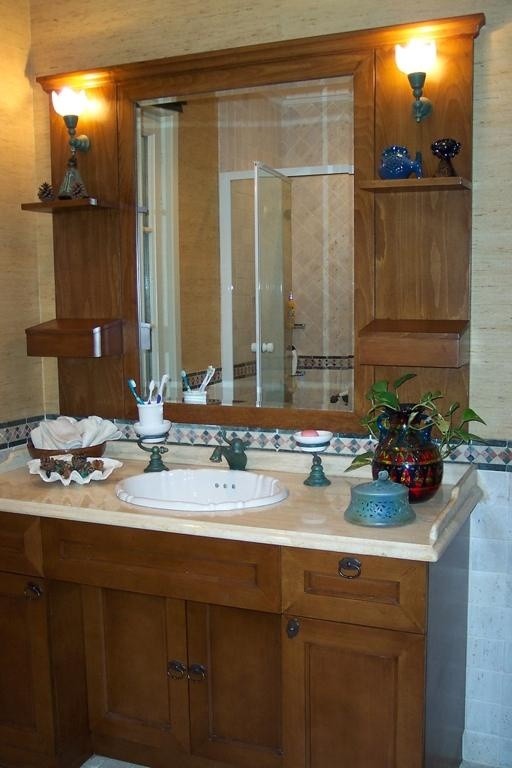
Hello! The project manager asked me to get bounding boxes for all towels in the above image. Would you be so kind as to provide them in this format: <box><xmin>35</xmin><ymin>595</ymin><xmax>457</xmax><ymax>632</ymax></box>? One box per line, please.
<box><xmin>30</xmin><ymin>414</ymin><xmax>123</xmax><ymax>450</ymax></box>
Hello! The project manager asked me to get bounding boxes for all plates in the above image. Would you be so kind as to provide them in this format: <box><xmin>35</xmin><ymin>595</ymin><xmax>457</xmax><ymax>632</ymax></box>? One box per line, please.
<box><xmin>25</xmin><ymin>453</ymin><xmax>123</xmax><ymax>486</ymax></box>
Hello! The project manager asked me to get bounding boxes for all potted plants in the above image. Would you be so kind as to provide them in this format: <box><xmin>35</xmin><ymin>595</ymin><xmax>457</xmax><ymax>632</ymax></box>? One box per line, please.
<box><xmin>341</xmin><ymin>372</ymin><xmax>486</xmax><ymax>503</ymax></box>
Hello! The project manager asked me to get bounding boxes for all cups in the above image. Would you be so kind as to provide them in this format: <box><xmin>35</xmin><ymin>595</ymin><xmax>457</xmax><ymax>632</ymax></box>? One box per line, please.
<box><xmin>136</xmin><ymin>401</ymin><xmax>163</xmax><ymax>425</ymax></box>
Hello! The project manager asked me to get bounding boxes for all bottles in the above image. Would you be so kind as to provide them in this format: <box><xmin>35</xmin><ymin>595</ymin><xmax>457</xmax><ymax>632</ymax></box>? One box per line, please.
<box><xmin>378</xmin><ymin>144</ymin><xmax>424</xmax><ymax>180</ymax></box>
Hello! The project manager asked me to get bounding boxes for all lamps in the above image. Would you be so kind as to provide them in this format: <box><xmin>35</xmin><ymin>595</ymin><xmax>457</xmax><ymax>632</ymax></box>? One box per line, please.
<box><xmin>392</xmin><ymin>38</ymin><xmax>437</xmax><ymax>125</ymax></box>
<box><xmin>53</xmin><ymin>87</ymin><xmax>91</xmax><ymax>158</ymax></box>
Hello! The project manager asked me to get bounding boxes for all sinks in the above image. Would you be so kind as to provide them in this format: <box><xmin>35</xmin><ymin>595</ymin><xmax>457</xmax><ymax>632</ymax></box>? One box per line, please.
<box><xmin>115</xmin><ymin>466</ymin><xmax>289</xmax><ymax>513</ymax></box>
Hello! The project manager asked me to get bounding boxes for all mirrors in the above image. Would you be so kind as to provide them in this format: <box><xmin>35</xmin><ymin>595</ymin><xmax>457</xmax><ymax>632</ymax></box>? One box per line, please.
<box><xmin>116</xmin><ymin>48</ymin><xmax>375</xmax><ymax>437</ymax></box>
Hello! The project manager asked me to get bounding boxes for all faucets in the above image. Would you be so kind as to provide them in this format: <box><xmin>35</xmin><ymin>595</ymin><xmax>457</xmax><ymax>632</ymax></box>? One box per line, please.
<box><xmin>209</xmin><ymin>429</ymin><xmax>248</xmax><ymax>470</ymax></box>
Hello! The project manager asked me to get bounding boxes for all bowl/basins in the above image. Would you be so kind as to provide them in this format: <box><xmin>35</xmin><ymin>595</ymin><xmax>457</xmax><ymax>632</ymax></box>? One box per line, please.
<box><xmin>294</xmin><ymin>429</ymin><xmax>333</xmax><ymax>453</ymax></box>
<box><xmin>134</xmin><ymin>420</ymin><xmax>172</xmax><ymax>443</ymax></box>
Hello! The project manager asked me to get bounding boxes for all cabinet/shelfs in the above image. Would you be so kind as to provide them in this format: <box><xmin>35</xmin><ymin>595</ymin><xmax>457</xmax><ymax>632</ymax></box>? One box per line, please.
<box><xmin>44</xmin><ymin>522</ymin><xmax>281</xmax><ymax>768</ymax></box>
<box><xmin>21</xmin><ymin>195</ymin><xmax>123</xmax><ymax>359</ymax></box>
<box><xmin>0</xmin><ymin>516</ymin><xmax>93</xmax><ymax>766</ymax></box>
<box><xmin>282</xmin><ymin>548</ymin><xmax>428</xmax><ymax>768</ymax></box>
<box><xmin>359</xmin><ymin>177</ymin><xmax>468</xmax><ymax>370</ymax></box>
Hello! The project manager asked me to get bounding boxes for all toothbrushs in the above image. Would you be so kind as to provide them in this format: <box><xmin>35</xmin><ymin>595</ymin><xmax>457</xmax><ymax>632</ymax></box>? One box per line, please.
<box><xmin>127</xmin><ymin>378</ymin><xmax>144</xmax><ymax>404</ymax></box>
<box><xmin>147</xmin><ymin>379</ymin><xmax>154</xmax><ymax>404</ymax></box>
<box><xmin>157</xmin><ymin>374</ymin><xmax>170</xmax><ymax>403</ymax></box>
<box><xmin>199</xmin><ymin>365</ymin><xmax>215</xmax><ymax>392</ymax></box>
<box><xmin>180</xmin><ymin>370</ymin><xmax>191</xmax><ymax>392</ymax></box>
